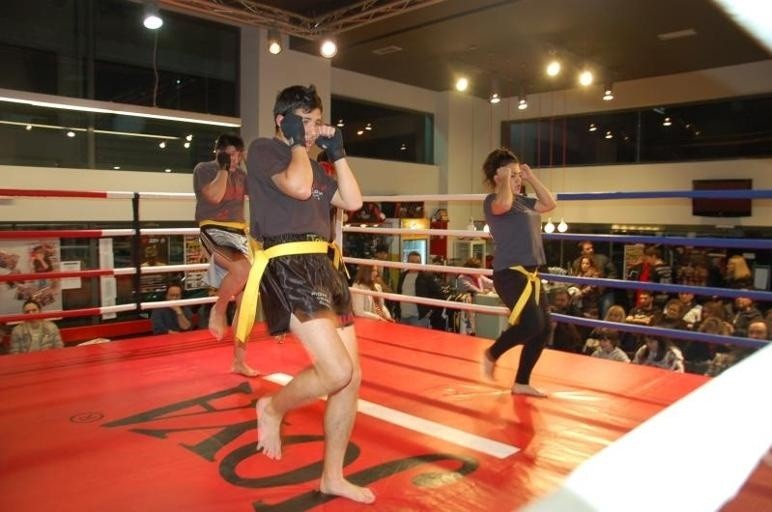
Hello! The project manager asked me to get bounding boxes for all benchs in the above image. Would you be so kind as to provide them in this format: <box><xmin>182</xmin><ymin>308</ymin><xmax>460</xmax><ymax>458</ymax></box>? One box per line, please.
<box><xmin>0</xmin><ymin>313</ymin><xmax>200</xmax><ymax>356</ymax></box>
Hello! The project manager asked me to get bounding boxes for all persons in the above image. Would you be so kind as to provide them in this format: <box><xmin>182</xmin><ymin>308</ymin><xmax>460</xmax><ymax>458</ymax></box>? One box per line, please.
<box><xmin>247</xmin><ymin>84</ymin><xmax>376</xmax><ymax>504</ymax></box>
<box><xmin>351</xmin><ymin>240</ymin><xmax>772</xmax><ymax>379</ymax></box>
<box><xmin>482</xmin><ymin>149</ymin><xmax>556</xmax><ymax>399</ymax></box>
<box><xmin>9</xmin><ymin>299</ymin><xmax>64</xmax><ymax>354</ymax></box>
<box><xmin>193</xmin><ymin>133</ymin><xmax>262</xmax><ymax>377</ymax></box>
<box><xmin>150</xmin><ymin>283</ymin><xmax>192</xmax><ymax>335</ymax></box>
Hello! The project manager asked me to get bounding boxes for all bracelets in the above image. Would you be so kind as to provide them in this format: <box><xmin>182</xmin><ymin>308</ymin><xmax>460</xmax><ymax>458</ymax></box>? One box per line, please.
<box><xmin>176</xmin><ymin>312</ymin><xmax>184</xmax><ymax>316</ymax></box>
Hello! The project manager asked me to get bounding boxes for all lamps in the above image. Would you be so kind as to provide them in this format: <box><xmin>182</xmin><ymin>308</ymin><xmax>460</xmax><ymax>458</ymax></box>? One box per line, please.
<box><xmin>543</xmin><ymin>47</ymin><xmax>614</xmax><ymax>101</ymax></box>
<box><xmin>453</xmin><ymin>67</ymin><xmax>528</xmax><ymax>110</ymax></box>
<box><xmin>337</xmin><ymin>119</ymin><xmax>407</xmax><ymax>151</ymax></box>
<box><xmin>266</xmin><ymin>28</ymin><xmax>282</xmax><ymax>55</ymax></box>
<box><xmin>0</xmin><ymin>117</ymin><xmax>193</xmax><ymax>151</ymax></box>
<box><xmin>318</xmin><ymin>35</ymin><xmax>338</xmax><ymax>59</ymax></box>
<box><xmin>465</xmin><ymin>216</ymin><xmax>568</xmax><ymax>235</ymax></box>
<box><xmin>589</xmin><ymin>117</ymin><xmax>671</xmax><ymax>139</ymax></box>
<box><xmin>143</xmin><ymin>1</ymin><xmax>163</xmax><ymax>29</ymax></box>
